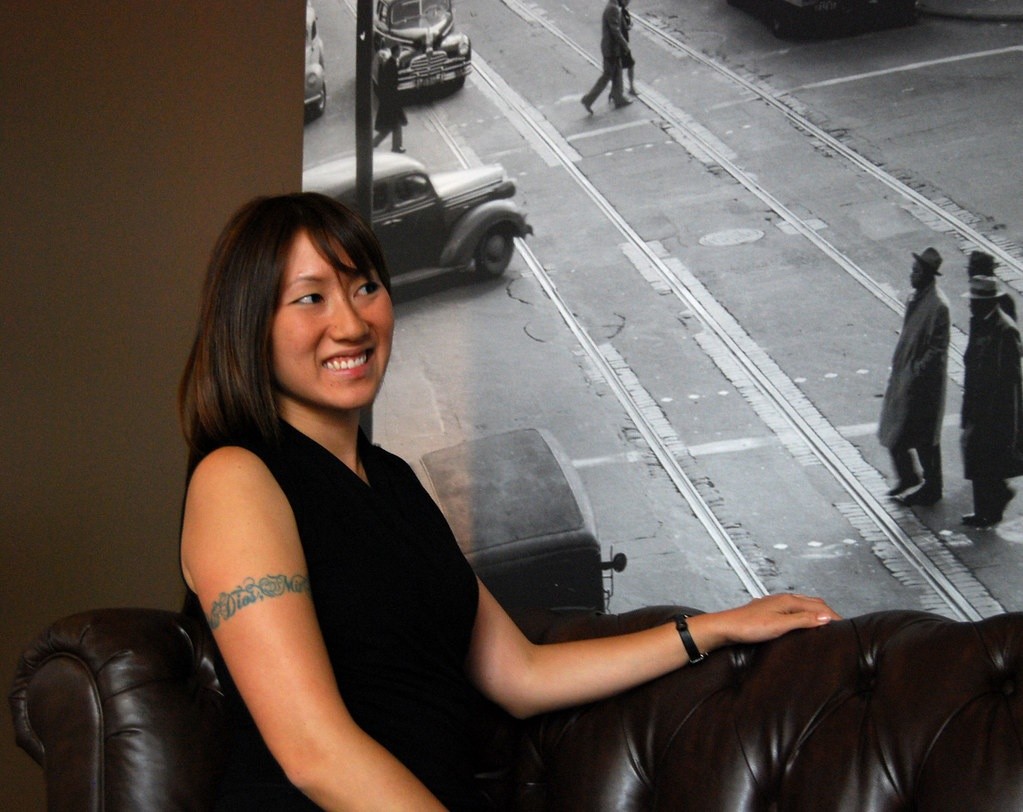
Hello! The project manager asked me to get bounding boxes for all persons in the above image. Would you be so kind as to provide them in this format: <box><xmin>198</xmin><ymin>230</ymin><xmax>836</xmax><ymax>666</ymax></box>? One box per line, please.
<box><xmin>581</xmin><ymin>0</ymin><xmax>640</xmax><ymax>114</ymax></box>
<box><xmin>373</xmin><ymin>44</ymin><xmax>407</xmax><ymax>152</ymax></box>
<box><xmin>178</xmin><ymin>193</ymin><xmax>842</xmax><ymax>811</ymax></box>
<box><xmin>961</xmin><ymin>252</ymin><xmax>1023</xmax><ymax>526</ymax></box>
<box><xmin>877</xmin><ymin>247</ymin><xmax>952</xmax><ymax>503</ymax></box>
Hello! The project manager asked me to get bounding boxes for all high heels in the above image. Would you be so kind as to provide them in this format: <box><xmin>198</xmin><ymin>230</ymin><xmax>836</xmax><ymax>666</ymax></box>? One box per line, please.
<box><xmin>608</xmin><ymin>93</ymin><xmax>626</xmax><ymax>103</ymax></box>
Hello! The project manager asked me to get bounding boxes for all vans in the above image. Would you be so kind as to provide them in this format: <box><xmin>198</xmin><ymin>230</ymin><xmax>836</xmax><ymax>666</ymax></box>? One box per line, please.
<box><xmin>417</xmin><ymin>426</ymin><xmax>627</xmax><ymax>646</ymax></box>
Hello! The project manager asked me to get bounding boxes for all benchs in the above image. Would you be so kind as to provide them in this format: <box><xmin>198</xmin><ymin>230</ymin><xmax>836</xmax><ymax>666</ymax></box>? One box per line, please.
<box><xmin>11</xmin><ymin>608</ymin><xmax>1021</xmax><ymax>812</ymax></box>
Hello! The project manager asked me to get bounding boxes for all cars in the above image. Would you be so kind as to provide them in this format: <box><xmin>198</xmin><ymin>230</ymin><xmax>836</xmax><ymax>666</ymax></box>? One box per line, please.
<box><xmin>370</xmin><ymin>1</ymin><xmax>472</xmax><ymax>103</ymax></box>
<box><xmin>303</xmin><ymin>2</ymin><xmax>328</xmax><ymax>121</ymax></box>
<box><xmin>301</xmin><ymin>146</ymin><xmax>533</xmax><ymax>296</ymax></box>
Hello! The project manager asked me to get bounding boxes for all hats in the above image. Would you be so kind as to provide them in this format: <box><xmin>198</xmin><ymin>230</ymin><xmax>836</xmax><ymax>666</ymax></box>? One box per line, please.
<box><xmin>911</xmin><ymin>246</ymin><xmax>945</xmax><ymax>277</ymax></box>
<box><xmin>959</xmin><ymin>275</ymin><xmax>1008</xmax><ymax>299</ymax></box>
<box><xmin>962</xmin><ymin>249</ymin><xmax>1002</xmax><ymax>270</ymax></box>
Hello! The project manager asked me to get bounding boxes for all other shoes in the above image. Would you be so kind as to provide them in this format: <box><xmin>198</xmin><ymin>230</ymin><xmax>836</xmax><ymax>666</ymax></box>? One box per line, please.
<box><xmin>985</xmin><ymin>491</ymin><xmax>1017</xmax><ymax>525</ymax></box>
<box><xmin>887</xmin><ymin>478</ymin><xmax>921</xmax><ymax>495</ymax></box>
<box><xmin>615</xmin><ymin>100</ymin><xmax>634</xmax><ymax>108</ymax></box>
<box><xmin>582</xmin><ymin>97</ymin><xmax>594</xmax><ymax>114</ymax></box>
<box><xmin>906</xmin><ymin>483</ymin><xmax>942</xmax><ymax>506</ymax></box>
<box><xmin>963</xmin><ymin>514</ymin><xmax>984</xmax><ymax>526</ymax></box>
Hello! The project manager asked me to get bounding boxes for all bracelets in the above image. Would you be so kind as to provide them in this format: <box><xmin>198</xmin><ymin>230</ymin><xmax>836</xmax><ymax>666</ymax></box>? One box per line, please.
<box><xmin>667</xmin><ymin>613</ymin><xmax>704</xmax><ymax>664</ymax></box>
